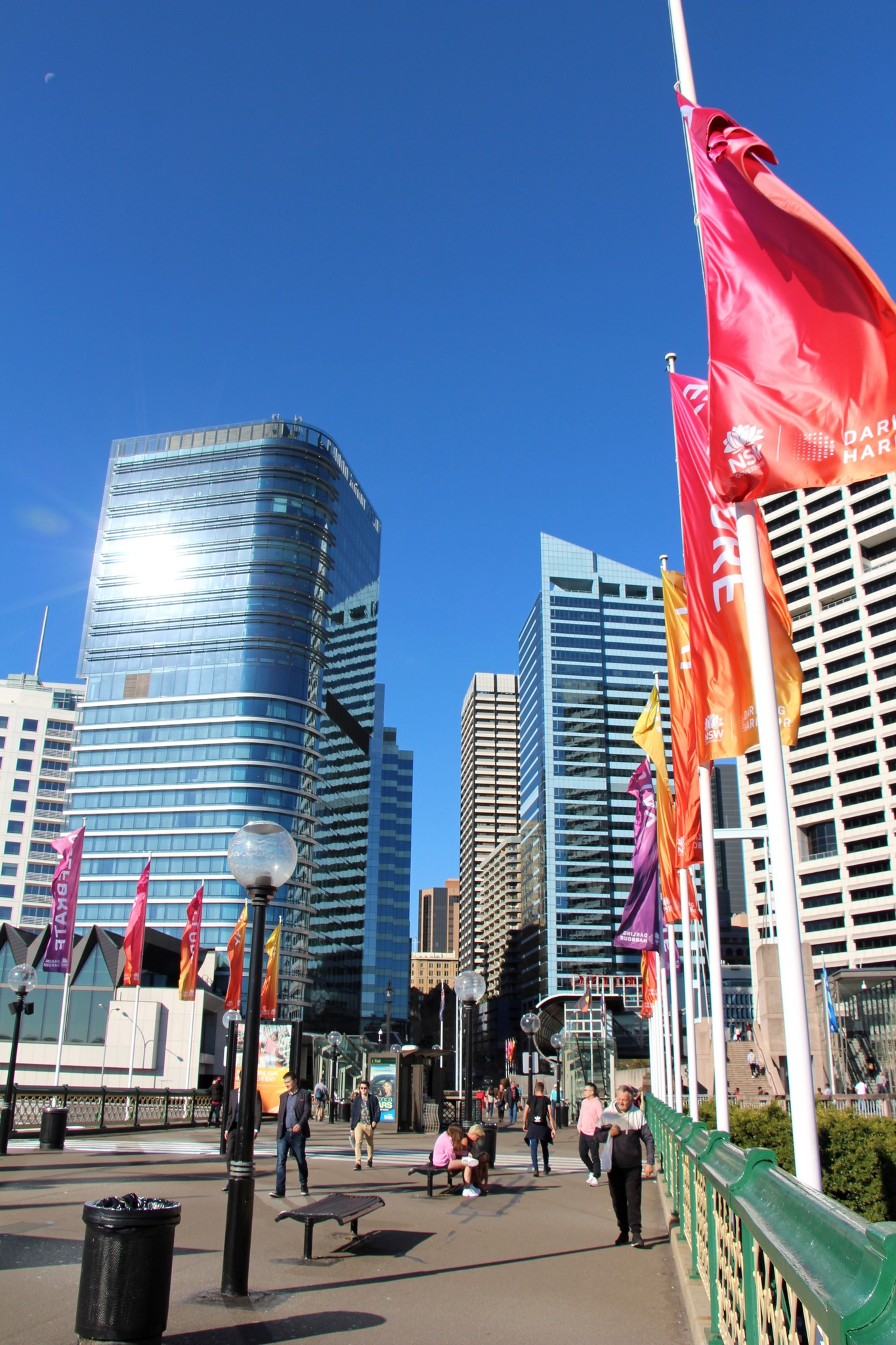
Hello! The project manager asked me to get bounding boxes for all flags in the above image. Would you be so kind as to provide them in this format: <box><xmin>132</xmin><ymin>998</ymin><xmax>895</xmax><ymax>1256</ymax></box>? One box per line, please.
<box><xmin>823</xmin><ymin>962</ymin><xmax>839</xmax><ymax>1033</ymax></box>
<box><xmin>44</xmin><ymin>826</ymin><xmax>281</xmax><ymax>1022</ymax></box>
<box><xmin>671</xmin><ymin>80</ymin><xmax>896</xmax><ymax>498</ymax></box>
<box><xmin>611</xmin><ymin>375</ymin><xmax>802</xmax><ymax>1023</ymax></box>
<box><xmin>505</xmin><ymin>1037</ymin><xmax>517</xmax><ymax>1064</ymax></box>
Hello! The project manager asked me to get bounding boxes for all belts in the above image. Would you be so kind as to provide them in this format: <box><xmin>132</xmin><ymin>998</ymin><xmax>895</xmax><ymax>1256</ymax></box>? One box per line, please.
<box><xmin>286</xmin><ymin>1128</ymin><xmax>293</xmax><ymax>1132</ymax></box>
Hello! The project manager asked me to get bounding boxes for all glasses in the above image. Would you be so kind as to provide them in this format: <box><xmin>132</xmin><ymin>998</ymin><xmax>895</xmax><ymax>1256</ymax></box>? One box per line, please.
<box><xmin>584</xmin><ymin>1088</ymin><xmax>594</xmax><ymax>1093</ymax></box>
<box><xmin>361</xmin><ymin>1087</ymin><xmax>367</xmax><ymax>1089</ymax></box>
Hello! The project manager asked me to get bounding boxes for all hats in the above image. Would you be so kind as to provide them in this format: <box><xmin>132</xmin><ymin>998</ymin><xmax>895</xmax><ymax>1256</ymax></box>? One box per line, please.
<box><xmin>554</xmin><ymin>1084</ymin><xmax>557</xmax><ymax>1087</ymax></box>
<box><xmin>321</xmin><ymin>1078</ymin><xmax>326</xmax><ymax>1082</ymax></box>
<box><xmin>217</xmin><ymin>1077</ymin><xmax>222</xmax><ymax>1081</ymax></box>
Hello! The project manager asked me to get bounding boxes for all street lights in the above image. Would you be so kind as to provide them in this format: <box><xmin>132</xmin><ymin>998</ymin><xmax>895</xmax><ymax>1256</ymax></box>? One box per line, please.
<box><xmin>520</xmin><ymin>1012</ymin><xmax>540</xmax><ymax>1098</ymax></box>
<box><xmin>382</xmin><ymin>979</ymin><xmax>395</xmax><ymax>1052</ymax></box>
<box><xmin>7</xmin><ymin>962</ymin><xmax>38</xmax><ymax>1109</ymax></box>
<box><xmin>328</xmin><ymin>1031</ymin><xmax>343</xmax><ymax>1124</ymax></box>
<box><xmin>453</xmin><ymin>970</ymin><xmax>486</xmax><ymax>1135</ymax></box>
<box><xmin>98</xmin><ymin>1004</ymin><xmax>120</xmax><ymax>1090</ymax></box>
<box><xmin>580</xmin><ymin>1051</ymin><xmax>591</xmax><ymax>1088</ymax></box>
<box><xmin>550</xmin><ymin>1033</ymin><xmax>565</xmax><ymax>1130</ymax></box>
<box><xmin>220</xmin><ymin>819</ymin><xmax>299</xmax><ymax>1296</ymax></box>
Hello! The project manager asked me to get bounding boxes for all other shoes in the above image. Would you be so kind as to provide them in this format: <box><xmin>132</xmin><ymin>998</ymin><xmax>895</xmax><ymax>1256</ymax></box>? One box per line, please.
<box><xmin>470</xmin><ymin>1184</ymin><xmax>481</xmax><ymax>1193</ymax></box>
<box><xmin>222</xmin><ymin>1187</ymin><xmax>228</xmax><ymax>1191</ymax></box>
<box><xmin>353</xmin><ymin>1163</ymin><xmax>362</xmax><ymax>1170</ymax></box>
<box><xmin>366</xmin><ymin>1159</ymin><xmax>373</xmax><ymax>1168</ymax></box>
<box><xmin>499</xmin><ymin>1119</ymin><xmax>503</xmax><ymax>1123</ymax></box>
<box><xmin>461</xmin><ymin>1156</ymin><xmax>478</xmax><ymax>1166</ymax></box>
<box><xmin>510</xmin><ymin>1121</ymin><xmax>517</xmax><ymax>1124</ymax></box>
<box><xmin>615</xmin><ymin>1232</ymin><xmax>645</xmax><ymax>1248</ymax></box>
<box><xmin>534</xmin><ymin>1166</ymin><xmax>552</xmax><ymax>1177</ymax></box>
<box><xmin>463</xmin><ymin>1187</ymin><xmax>479</xmax><ymax>1197</ymax></box>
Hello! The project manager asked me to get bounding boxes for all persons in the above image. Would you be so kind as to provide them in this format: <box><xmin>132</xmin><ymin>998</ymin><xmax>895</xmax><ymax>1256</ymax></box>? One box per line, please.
<box><xmin>550</xmin><ymin>1084</ymin><xmax>557</xmax><ymax>1120</ymax></box>
<box><xmin>758</xmin><ymin>1086</ymin><xmax>766</xmax><ymax>1103</ymax></box>
<box><xmin>476</xmin><ymin>1081</ymin><xmax>521</xmax><ymax>1125</ymax></box>
<box><xmin>745</xmin><ymin>1020</ymin><xmax>752</xmax><ymax>1041</ymax></box>
<box><xmin>461</xmin><ymin>1123</ymin><xmax>489</xmax><ymax>1192</ymax></box>
<box><xmin>577</xmin><ymin>1082</ymin><xmax>604</xmax><ymax>1186</ymax></box>
<box><xmin>313</xmin><ymin>1078</ymin><xmax>330</xmax><ymax>1121</ymax></box>
<box><xmin>733</xmin><ymin>1088</ymin><xmax>741</xmax><ymax>1096</ymax></box>
<box><xmin>263</xmin><ymin>1026</ymin><xmax>278</xmax><ymax>1069</ymax></box>
<box><xmin>378</xmin><ymin>1079</ymin><xmax>393</xmax><ymax>1098</ymax></box>
<box><xmin>350</xmin><ymin>1081</ymin><xmax>381</xmax><ymax>1171</ymax></box>
<box><xmin>594</xmin><ymin>1086</ymin><xmax>655</xmax><ymax>1246</ymax></box>
<box><xmin>523</xmin><ymin>1082</ymin><xmax>556</xmax><ymax>1177</ymax></box>
<box><xmin>735</xmin><ymin>1024</ymin><xmax>742</xmax><ymax>1042</ymax></box>
<box><xmin>348</xmin><ymin>1083</ymin><xmax>359</xmax><ymax>1122</ymax></box>
<box><xmin>207</xmin><ymin>1077</ymin><xmax>223</xmax><ymax>1128</ymax></box>
<box><xmin>269</xmin><ymin>1072</ymin><xmax>311</xmax><ymax>1197</ymax></box>
<box><xmin>747</xmin><ymin>1049</ymin><xmax>758</xmax><ymax>1078</ymax></box>
<box><xmin>433</xmin><ymin>1123</ymin><xmax>481</xmax><ymax>1197</ymax></box>
<box><xmin>222</xmin><ymin>1072</ymin><xmax>262</xmax><ymax>1192</ymax></box>
<box><xmin>817</xmin><ymin>1053</ymin><xmax>886</xmax><ymax>1112</ymax></box>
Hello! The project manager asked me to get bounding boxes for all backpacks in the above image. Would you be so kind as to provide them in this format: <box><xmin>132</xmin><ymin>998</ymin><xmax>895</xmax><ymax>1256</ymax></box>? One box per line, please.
<box><xmin>316</xmin><ymin>1087</ymin><xmax>325</xmax><ymax>1100</ymax></box>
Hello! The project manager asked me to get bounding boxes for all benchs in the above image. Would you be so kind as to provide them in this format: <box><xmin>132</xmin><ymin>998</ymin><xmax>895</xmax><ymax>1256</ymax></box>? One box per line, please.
<box><xmin>406</xmin><ymin>1161</ymin><xmax>457</xmax><ymax>1199</ymax></box>
<box><xmin>273</xmin><ymin>1189</ymin><xmax>387</xmax><ymax>1265</ymax></box>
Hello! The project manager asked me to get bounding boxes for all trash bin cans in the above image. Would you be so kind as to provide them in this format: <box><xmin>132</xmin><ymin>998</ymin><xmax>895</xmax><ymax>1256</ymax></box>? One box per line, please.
<box><xmin>74</xmin><ymin>1197</ymin><xmax>182</xmax><ymax>1345</ymax></box>
<box><xmin>39</xmin><ymin>1107</ymin><xmax>69</xmax><ymax>1149</ymax></box>
<box><xmin>473</xmin><ymin>1124</ymin><xmax>498</xmax><ymax>1169</ymax></box>
<box><xmin>556</xmin><ymin>1106</ymin><xmax>569</xmax><ymax>1127</ymax></box>
<box><xmin>338</xmin><ymin>1102</ymin><xmax>351</xmax><ymax>1122</ymax></box>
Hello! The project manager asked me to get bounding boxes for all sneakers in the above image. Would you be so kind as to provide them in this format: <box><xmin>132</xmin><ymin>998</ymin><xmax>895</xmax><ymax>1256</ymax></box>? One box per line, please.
<box><xmin>585</xmin><ymin>1172</ymin><xmax>599</xmax><ymax>1187</ymax></box>
<box><xmin>270</xmin><ymin>1191</ymin><xmax>285</xmax><ymax>1198</ymax></box>
<box><xmin>301</xmin><ymin>1185</ymin><xmax>309</xmax><ymax>1195</ymax></box>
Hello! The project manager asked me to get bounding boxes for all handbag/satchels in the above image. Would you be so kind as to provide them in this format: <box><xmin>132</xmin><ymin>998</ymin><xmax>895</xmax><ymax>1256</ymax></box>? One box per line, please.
<box><xmin>495</xmin><ymin>1100</ymin><xmax>503</xmax><ymax>1107</ymax></box>
<box><xmin>601</xmin><ymin>1133</ymin><xmax>613</xmax><ymax>1173</ymax></box>
